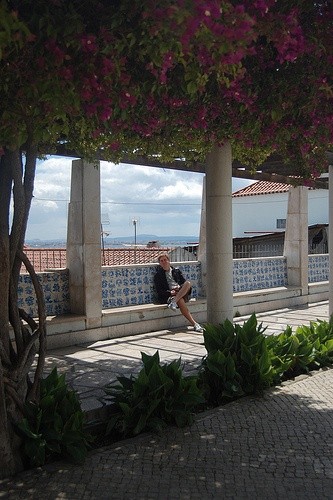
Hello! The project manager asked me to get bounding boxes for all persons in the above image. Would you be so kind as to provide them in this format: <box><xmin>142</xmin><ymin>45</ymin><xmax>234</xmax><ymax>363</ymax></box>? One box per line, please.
<box><xmin>153</xmin><ymin>253</ymin><xmax>204</xmax><ymax>332</ymax></box>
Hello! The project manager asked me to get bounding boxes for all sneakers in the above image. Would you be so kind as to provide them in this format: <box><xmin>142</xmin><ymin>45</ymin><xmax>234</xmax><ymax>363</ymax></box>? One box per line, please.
<box><xmin>194</xmin><ymin>323</ymin><xmax>203</xmax><ymax>332</ymax></box>
<box><xmin>168</xmin><ymin>302</ymin><xmax>176</xmax><ymax>311</ymax></box>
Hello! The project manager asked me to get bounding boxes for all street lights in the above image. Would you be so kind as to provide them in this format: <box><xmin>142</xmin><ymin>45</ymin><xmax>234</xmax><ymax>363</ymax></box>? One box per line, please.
<box><xmin>133</xmin><ymin>220</ymin><xmax>136</xmax><ymax>244</ymax></box>
